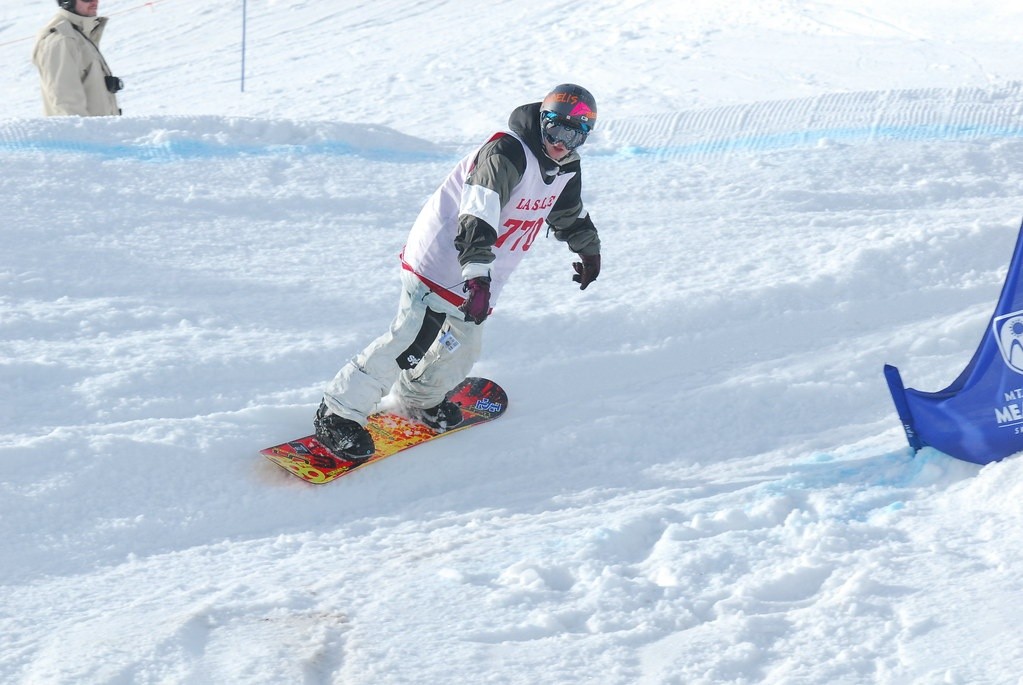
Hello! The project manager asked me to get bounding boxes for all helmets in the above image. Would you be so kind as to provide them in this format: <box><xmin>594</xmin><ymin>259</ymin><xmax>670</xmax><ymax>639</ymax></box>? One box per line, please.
<box><xmin>539</xmin><ymin>84</ymin><xmax>597</xmax><ymax>130</ymax></box>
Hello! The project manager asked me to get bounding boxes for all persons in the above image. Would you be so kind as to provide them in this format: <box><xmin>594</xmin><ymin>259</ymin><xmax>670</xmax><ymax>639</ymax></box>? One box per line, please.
<box><xmin>316</xmin><ymin>83</ymin><xmax>600</xmax><ymax>464</ymax></box>
<box><xmin>35</xmin><ymin>0</ymin><xmax>122</xmax><ymax>117</ymax></box>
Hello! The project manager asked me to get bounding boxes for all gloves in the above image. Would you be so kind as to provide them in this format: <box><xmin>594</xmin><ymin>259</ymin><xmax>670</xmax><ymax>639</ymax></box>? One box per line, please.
<box><xmin>457</xmin><ymin>276</ymin><xmax>491</xmax><ymax>325</ymax></box>
<box><xmin>571</xmin><ymin>253</ymin><xmax>601</xmax><ymax>290</ymax></box>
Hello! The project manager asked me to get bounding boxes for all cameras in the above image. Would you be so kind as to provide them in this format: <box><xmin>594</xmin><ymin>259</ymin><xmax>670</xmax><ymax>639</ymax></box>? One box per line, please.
<box><xmin>104</xmin><ymin>76</ymin><xmax>124</xmax><ymax>94</ymax></box>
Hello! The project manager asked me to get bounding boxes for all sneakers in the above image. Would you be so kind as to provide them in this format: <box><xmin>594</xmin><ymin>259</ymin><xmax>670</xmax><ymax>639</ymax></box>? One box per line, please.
<box><xmin>313</xmin><ymin>397</ymin><xmax>375</xmax><ymax>459</ymax></box>
<box><xmin>388</xmin><ymin>390</ymin><xmax>464</xmax><ymax>429</ymax></box>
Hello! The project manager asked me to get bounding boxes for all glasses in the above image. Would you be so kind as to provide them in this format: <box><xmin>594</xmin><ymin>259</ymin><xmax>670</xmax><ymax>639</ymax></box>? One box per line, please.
<box><xmin>541</xmin><ymin>116</ymin><xmax>587</xmax><ymax>150</ymax></box>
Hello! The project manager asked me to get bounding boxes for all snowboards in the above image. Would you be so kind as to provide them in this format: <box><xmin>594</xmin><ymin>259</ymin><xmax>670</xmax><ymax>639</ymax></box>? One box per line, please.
<box><xmin>257</xmin><ymin>376</ymin><xmax>510</xmax><ymax>485</ymax></box>
